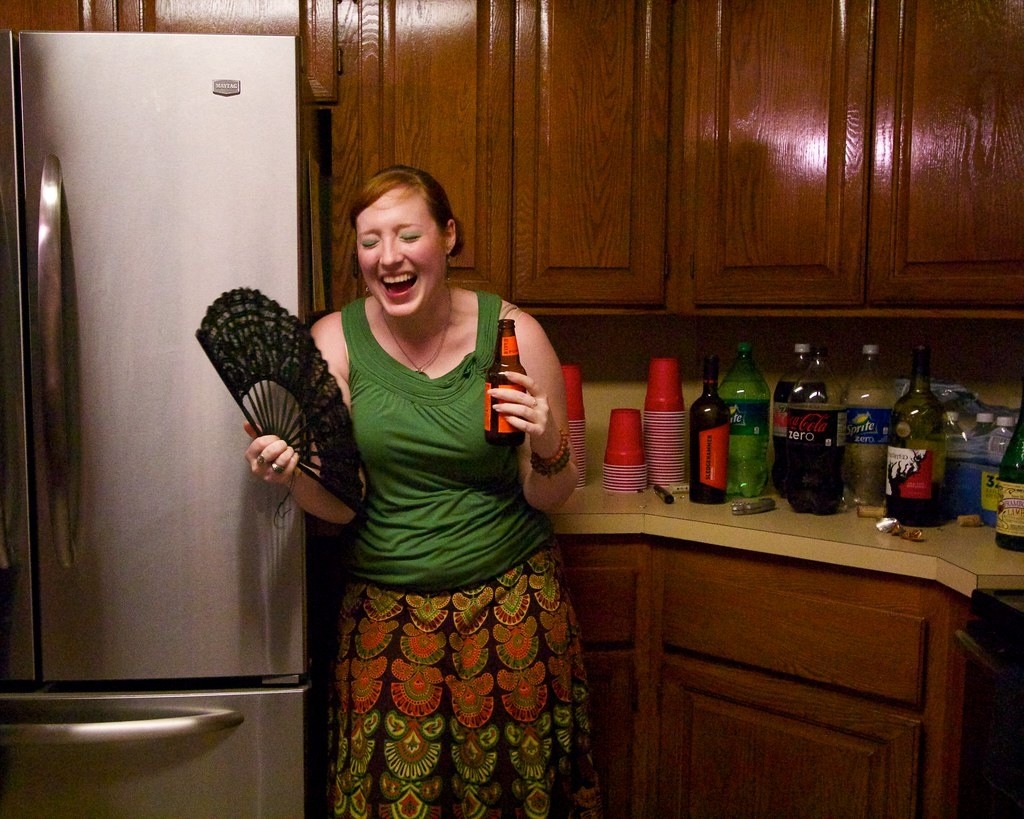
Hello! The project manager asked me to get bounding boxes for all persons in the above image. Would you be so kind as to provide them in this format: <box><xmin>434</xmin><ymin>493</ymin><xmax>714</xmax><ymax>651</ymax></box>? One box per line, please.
<box><xmin>243</xmin><ymin>164</ymin><xmax>603</xmax><ymax>819</ymax></box>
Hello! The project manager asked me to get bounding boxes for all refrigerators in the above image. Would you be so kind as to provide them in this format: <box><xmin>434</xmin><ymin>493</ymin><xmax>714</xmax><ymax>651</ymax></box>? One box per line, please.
<box><xmin>0</xmin><ymin>28</ymin><xmax>309</xmax><ymax>819</ymax></box>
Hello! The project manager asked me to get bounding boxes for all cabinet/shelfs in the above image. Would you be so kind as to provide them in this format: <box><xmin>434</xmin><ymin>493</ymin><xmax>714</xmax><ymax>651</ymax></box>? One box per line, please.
<box><xmin>0</xmin><ymin>1</ymin><xmax>1024</xmax><ymax>325</ymax></box>
<box><xmin>547</xmin><ymin>539</ymin><xmax>1024</xmax><ymax>819</ymax></box>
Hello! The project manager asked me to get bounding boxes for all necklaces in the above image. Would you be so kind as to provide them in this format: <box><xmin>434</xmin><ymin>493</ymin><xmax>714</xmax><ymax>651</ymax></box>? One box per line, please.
<box><xmin>382</xmin><ymin>282</ymin><xmax>452</xmax><ymax>377</ymax></box>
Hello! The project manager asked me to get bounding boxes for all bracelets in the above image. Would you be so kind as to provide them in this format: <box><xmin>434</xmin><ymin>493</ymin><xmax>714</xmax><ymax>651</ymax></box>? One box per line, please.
<box><xmin>530</xmin><ymin>425</ymin><xmax>571</xmax><ymax>478</ymax></box>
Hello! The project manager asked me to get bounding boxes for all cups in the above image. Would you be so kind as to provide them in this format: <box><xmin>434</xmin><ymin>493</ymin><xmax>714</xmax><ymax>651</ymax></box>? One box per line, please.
<box><xmin>603</xmin><ymin>409</ymin><xmax>648</xmax><ymax>492</ymax></box>
<box><xmin>644</xmin><ymin>355</ymin><xmax>686</xmax><ymax>485</ymax></box>
<box><xmin>559</xmin><ymin>362</ymin><xmax>586</xmax><ymax>488</ymax></box>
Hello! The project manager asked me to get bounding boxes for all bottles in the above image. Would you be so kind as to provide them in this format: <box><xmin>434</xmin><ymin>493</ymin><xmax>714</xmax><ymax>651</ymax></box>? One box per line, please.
<box><xmin>886</xmin><ymin>345</ymin><xmax>946</xmax><ymax>527</ymax></box>
<box><xmin>942</xmin><ymin>392</ymin><xmax>1020</xmax><ymax>527</ymax></box>
<box><xmin>995</xmin><ymin>385</ymin><xmax>1024</xmax><ymax>552</ymax></box>
<box><xmin>484</xmin><ymin>319</ymin><xmax>527</xmax><ymax>447</ymax></box>
<box><xmin>772</xmin><ymin>341</ymin><xmax>845</xmax><ymax>514</ymax></box>
<box><xmin>845</xmin><ymin>343</ymin><xmax>896</xmax><ymax>504</ymax></box>
<box><xmin>690</xmin><ymin>354</ymin><xmax>730</xmax><ymax>503</ymax></box>
<box><xmin>718</xmin><ymin>339</ymin><xmax>771</xmax><ymax>498</ymax></box>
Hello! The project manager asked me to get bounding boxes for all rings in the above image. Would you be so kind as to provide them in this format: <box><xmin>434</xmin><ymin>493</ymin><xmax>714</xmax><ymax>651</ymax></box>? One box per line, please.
<box><xmin>271</xmin><ymin>462</ymin><xmax>284</xmax><ymax>475</ymax></box>
<box><xmin>256</xmin><ymin>454</ymin><xmax>268</xmax><ymax>468</ymax></box>
<box><xmin>529</xmin><ymin>396</ymin><xmax>538</xmax><ymax>409</ymax></box>
